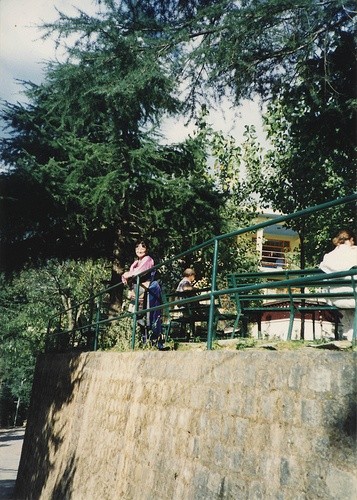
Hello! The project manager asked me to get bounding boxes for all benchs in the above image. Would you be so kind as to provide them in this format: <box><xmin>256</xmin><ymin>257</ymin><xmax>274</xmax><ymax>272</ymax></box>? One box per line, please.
<box><xmin>226</xmin><ymin>264</ymin><xmax>357</xmax><ymax>341</ymax></box>
<box><xmin>162</xmin><ymin>286</ymin><xmax>244</xmax><ymax>340</ymax></box>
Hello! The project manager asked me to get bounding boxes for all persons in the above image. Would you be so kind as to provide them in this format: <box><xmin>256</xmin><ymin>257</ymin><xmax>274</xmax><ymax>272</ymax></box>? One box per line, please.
<box><xmin>318</xmin><ymin>229</ymin><xmax>357</xmax><ymax>309</ymax></box>
<box><xmin>172</xmin><ymin>268</ymin><xmax>197</xmax><ymax>319</ymax></box>
<box><xmin>121</xmin><ymin>239</ymin><xmax>156</xmax><ymax>348</ymax></box>
